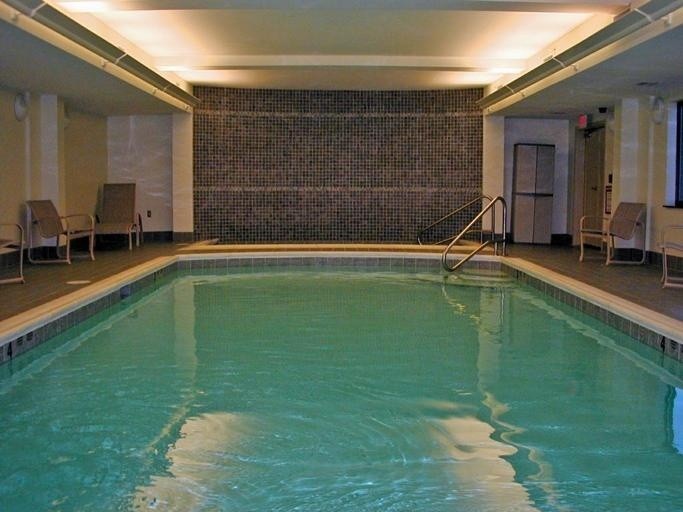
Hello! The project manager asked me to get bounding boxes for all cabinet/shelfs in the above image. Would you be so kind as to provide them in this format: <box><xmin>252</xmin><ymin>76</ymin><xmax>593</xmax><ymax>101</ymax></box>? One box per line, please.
<box><xmin>511</xmin><ymin>143</ymin><xmax>554</xmax><ymax>245</ymax></box>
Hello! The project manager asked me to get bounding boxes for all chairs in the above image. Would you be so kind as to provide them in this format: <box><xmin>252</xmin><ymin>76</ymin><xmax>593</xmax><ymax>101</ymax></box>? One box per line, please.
<box><xmin>1</xmin><ymin>183</ymin><xmax>142</xmax><ymax>286</ymax></box>
<box><xmin>577</xmin><ymin>202</ymin><xmax>682</xmax><ymax>292</ymax></box>
